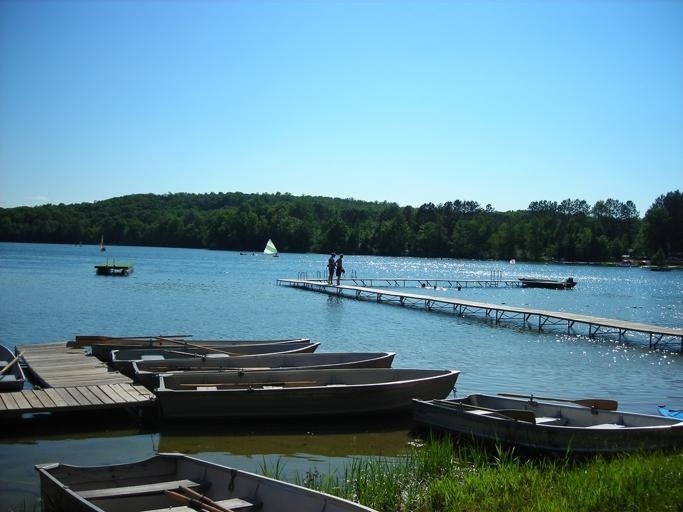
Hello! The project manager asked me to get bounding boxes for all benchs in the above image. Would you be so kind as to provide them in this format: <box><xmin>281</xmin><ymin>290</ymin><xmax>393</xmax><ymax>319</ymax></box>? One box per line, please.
<box><xmin>589</xmin><ymin>422</ymin><xmax>627</xmax><ymax>430</ymax></box>
<box><xmin>140</xmin><ymin>352</ymin><xmax>165</xmax><ymax>360</ymax></box>
<box><xmin>534</xmin><ymin>416</ymin><xmax>568</xmax><ymax>426</ymax></box>
<box><xmin>76</xmin><ymin>477</ymin><xmax>202</xmax><ymax>502</ymax></box>
<box><xmin>467</xmin><ymin>407</ymin><xmax>501</xmax><ymax>415</ymax></box>
<box><xmin>144</xmin><ymin>496</ymin><xmax>258</xmax><ymax>512</ymax></box>
<box><xmin>205</xmin><ymin>351</ymin><xmax>231</xmax><ymax>358</ymax></box>
<box><xmin>195</xmin><ymin>383</ymin><xmax>217</xmax><ymax>391</ymax></box>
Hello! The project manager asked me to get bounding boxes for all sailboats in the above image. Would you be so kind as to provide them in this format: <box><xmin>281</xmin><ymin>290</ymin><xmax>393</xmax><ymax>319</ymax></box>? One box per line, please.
<box><xmin>262</xmin><ymin>238</ymin><xmax>279</xmax><ymax>258</ymax></box>
<box><xmin>100</xmin><ymin>234</ymin><xmax>105</xmax><ymax>251</ymax></box>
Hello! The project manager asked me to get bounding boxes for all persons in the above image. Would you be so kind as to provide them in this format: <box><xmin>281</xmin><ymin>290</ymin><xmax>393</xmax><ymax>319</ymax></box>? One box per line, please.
<box><xmin>328</xmin><ymin>253</ymin><xmax>336</xmax><ymax>284</ymax></box>
<box><xmin>336</xmin><ymin>255</ymin><xmax>345</xmax><ymax>285</ymax></box>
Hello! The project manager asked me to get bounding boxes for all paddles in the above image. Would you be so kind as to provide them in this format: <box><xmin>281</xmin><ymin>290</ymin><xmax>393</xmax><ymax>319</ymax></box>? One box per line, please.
<box><xmin>155</xmin><ymin>336</ymin><xmax>242</xmax><ymax>355</ymax></box>
<box><xmin>434</xmin><ymin>399</ymin><xmax>534</xmax><ymax>423</ymax></box>
<box><xmin>498</xmin><ymin>393</ymin><xmax>617</xmax><ymax>411</ymax></box>
<box><xmin>181</xmin><ymin>381</ymin><xmax>316</xmax><ymax>386</ymax></box>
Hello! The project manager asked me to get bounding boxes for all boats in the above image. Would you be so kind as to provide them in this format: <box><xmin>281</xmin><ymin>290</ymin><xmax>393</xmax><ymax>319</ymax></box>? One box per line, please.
<box><xmin>31</xmin><ymin>451</ymin><xmax>380</xmax><ymax>512</ymax></box>
<box><xmin>239</xmin><ymin>252</ymin><xmax>246</xmax><ymax>255</ymax></box>
<box><xmin>92</xmin><ymin>259</ymin><xmax>136</xmax><ymax>276</ymax></box>
<box><xmin>518</xmin><ymin>276</ymin><xmax>577</xmax><ymax>290</ymax></box>
<box><xmin>409</xmin><ymin>392</ymin><xmax>683</xmax><ymax>453</ymax></box>
<box><xmin>655</xmin><ymin>403</ymin><xmax>683</xmax><ymax>421</ymax></box>
<box><xmin>248</xmin><ymin>253</ymin><xmax>259</xmax><ymax>256</ymax></box>
<box><xmin>0</xmin><ymin>344</ymin><xmax>25</xmax><ymax>391</ymax></box>
